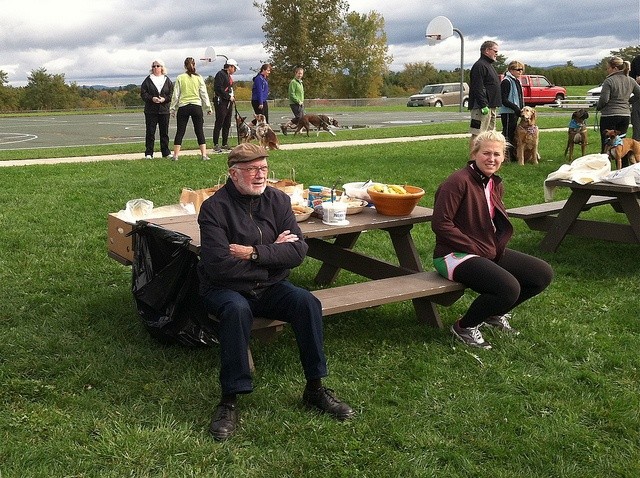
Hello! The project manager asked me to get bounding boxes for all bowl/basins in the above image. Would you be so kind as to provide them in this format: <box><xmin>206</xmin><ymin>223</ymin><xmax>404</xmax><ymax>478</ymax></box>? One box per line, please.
<box><xmin>341</xmin><ymin>181</ymin><xmax>375</xmax><ymax>197</ymax></box>
<box><xmin>368</xmin><ymin>184</ymin><xmax>426</xmax><ymax>216</ymax></box>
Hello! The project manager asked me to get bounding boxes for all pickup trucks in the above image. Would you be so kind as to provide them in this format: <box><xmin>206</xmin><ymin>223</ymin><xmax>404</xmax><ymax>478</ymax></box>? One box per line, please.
<box><xmin>497</xmin><ymin>74</ymin><xmax>566</xmax><ymax>106</ymax></box>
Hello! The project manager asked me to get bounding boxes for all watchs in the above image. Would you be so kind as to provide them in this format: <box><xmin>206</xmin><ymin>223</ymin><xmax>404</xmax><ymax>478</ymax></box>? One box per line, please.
<box><xmin>251</xmin><ymin>247</ymin><xmax>258</xmax><ymax>260</ymax></box>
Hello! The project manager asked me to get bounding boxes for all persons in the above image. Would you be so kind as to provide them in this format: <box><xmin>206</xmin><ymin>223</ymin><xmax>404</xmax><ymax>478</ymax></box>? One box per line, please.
<box><xmin>197</xmin><ymin>143</ymin><xmax>356</xmax><ymax>440</ymax></box>
<box><xmin>170</xmin><ymin>57</ymin><xmax>213</xmax><ymax>161</ymax></box>
<box><xmin>629</xmin><ymin>55</ymin><xmax>640</xmax><ymax>141</ymax></box>
<box><xmin>500</xmin><ymin>61</ymin><xmax>525</xmax><ymax>161</ymax></box>
<box><xmin>140</xmin><ymin>59</ymin><xmax>174</xmax><ymax>159</ymax></box>
<box><xmin>592</xmin><ymin>57</ymin><xmax>640</xmax><ymax>170</ymax></box>
<box><xmin>467</xmin><ymin>40</ymin><xmax>503</xmax><ymax>161</ymax></box>
<box><xmin>280</xmin><ymin>68</ymin><xmax>305</xmax><ymax>136</ymax></box>
<box><xmin>214</xmin><ymin>59</ymin><xmax>240</xmax><ymax>152</ymax></box>
<box><xmin>431</xmin><ymin>131</ymin><xmax>553</xmax><ymax>350</ymax></box>
<box><xmin>251</xmin><ymin>64</ymin><xmax>271</xmax><ymax>125</ymax></box>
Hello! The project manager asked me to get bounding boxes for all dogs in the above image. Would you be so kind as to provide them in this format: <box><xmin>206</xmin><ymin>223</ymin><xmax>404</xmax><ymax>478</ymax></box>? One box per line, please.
<box><xmin>235</xmin><ymin>114</ymin><xmax>259</xmax><ymax>144</ymax></box>
<box><xmin>514</xmin><ymin>105</ymin><xmax>541</xmax><ymax>165</ymax></box>
<box><xmin>602</xmin><ymin>128</ymin><xmax>639</xmax><ymax>169</ymax></box>
<box><xmin>288</xmin><ymin>113</ymin><xmax>339</xmax><ymax>137</ymax></box>
<box><xmin>564</xmin><ymin>108</ymin><xmax>589</xmax><ymax>164</ymax></box>
<box><xmin>253</xmin><ymin>113</ymin><xmax>281</xmax><ymax>150</ymax></box>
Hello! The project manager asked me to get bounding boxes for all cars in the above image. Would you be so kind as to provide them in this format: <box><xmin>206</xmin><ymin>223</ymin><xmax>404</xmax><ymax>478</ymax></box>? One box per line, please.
<box><xmin>586</xmin><ymin>81</ymin><xmax>635</xmax><ymax>107</ymax></box>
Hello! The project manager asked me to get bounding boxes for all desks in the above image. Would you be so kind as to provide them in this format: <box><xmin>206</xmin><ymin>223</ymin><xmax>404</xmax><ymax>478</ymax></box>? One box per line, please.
<box><xmin>523</xmin><ymin>176</ymin><xmax>640</xmax><ymax>255</ymax></box>
<box><xmin>150</xmin><ymin>192</ymin><xmax>445</xmax><ymax>331</ymax></box>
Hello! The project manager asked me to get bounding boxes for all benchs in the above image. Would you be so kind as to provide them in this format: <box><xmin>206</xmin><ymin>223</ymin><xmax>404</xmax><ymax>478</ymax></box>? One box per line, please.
<box><xmin>505</xmin><ymin>195</ymin><xmax>619</xmax><ymax>220</ymax></box>
<box><xmin>208</xmin><ymin>270</ymin><xmax>470</xmax><ymax>332</ymax></box>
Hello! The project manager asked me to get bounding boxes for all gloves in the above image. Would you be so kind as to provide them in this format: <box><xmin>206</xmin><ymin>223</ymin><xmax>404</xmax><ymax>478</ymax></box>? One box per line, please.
<box><xmin>481</xmin><ymin>106</ymin><xmax>489</xmax><ymax>115</ymax></box>
<box><xmin>514</xmin><ymin>105</ymin><xmax>522</xmax><ymax>117</ymax></box>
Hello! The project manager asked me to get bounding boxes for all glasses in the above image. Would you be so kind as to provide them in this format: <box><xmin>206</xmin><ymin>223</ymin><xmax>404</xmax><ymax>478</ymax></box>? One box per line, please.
<box><xmin>153</xmin><ymin>65</ymin><xmax>159</xmax><ymax>67</ymax></box>
<box><xmin>512</xmin><ymin>68</ymin><xmax>523</xmax><ymax>71</ymax></box>
<box><xmin>232</xmin><ymin>165</ymin><xmax>268</xmax><ymax>173</ymax></box>
<box><xmin>489</xmin><ymin>49</ymin><xmax>497</xmax><ymax>53</ymax></box>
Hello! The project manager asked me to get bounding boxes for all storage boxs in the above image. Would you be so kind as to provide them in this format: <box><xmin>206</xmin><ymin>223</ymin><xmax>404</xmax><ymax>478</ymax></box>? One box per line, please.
<box><xmin>108</xmin><ymin>204</ymin><xmax>195</xmax><ymax>266</ymax></box>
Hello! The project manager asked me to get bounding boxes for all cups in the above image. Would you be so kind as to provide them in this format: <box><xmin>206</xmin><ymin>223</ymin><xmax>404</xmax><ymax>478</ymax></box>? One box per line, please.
<box><xmin>321</xmin><ymin>202</ymin><xmax>348</xmax><ymax>225</ymax></box>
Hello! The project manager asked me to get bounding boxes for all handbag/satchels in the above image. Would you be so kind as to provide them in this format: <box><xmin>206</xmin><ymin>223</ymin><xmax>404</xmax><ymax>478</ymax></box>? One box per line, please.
<box><xmin>180</xmin><ymin>188</ymin><xmax>199</xmax><ymax>213</ymax></box>
<box><xmin>269</xmin><ymin>168</ymin><xmax>303</xmax><ymax>198</ymax></box>
<box><xmin>196</xmin><ymin>174</ymin><xmax>228</xmax><ymax>211</ymax></box>
<box><xmin>599</xmin><ymin>162</ymin><xmax>640</xmax><ymax>186</ymax></box>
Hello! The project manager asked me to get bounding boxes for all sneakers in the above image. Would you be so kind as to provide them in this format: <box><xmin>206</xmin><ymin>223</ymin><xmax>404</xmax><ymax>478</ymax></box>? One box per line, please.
<box><xmin>202</xmin><ymin>155</ymin><xmax>210</xmax><ymax>160</ymax></box>
<box><xmin>210</xmin><ymin>402</ymin><xmax>239</xmax><ymax>441</ymax></box>
<box><xmin>221</xmin><ymin>145</ymin><xmax>232</xmax><ymax>151</ymax></box>
<box><xmin>146</xmin><ymin>155</ymin><xmax>151</xmax><ymax>159</ymax></box>
<box><xmin>449</xmin><ymin>318</ymin><xmax>493</xmax><ymax>350</ymax></box>
<box><xmin>303</xmin><ymin>385</ymin><xmax>353</xmax><ymax>419</ymax></box>
<box><xmin>167</xmin><ymin>154</ymin><xmax>173</xmax><ymax>159</ymax></box>
<box><xmin>170</xmin><ymin>156</ymin><xmax>178</xmax><ymax>160</ymax></box>
<box><xmin>300</xmin><ymin>133</ymin><xmax>303</xmax><ymax>135</ymax></box>
<box><xmin>485</xmin><ymin>314</ymin><xmax>520</xmax><ymax>335</ymax></box>
<box><xmin>213</xmin><ymin>144</ymin><xmax>222</xmax><ymax>152</ymax></box>
<box><xmin>281</xmin><ymin>126</ymin><xmax>287</xmax><ymax>135</ymax></box>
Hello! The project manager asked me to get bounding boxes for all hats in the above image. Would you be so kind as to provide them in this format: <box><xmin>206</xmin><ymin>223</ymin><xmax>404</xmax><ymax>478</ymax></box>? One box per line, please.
<box><xmin>228</xmin><ymin>143</ymin><xmax>269</xmax><ymax>168</ymax></box>
<box><xmin>227</xmin><ymin>59</ymin><xmax>240</xmax><ymax>70</ymax></box>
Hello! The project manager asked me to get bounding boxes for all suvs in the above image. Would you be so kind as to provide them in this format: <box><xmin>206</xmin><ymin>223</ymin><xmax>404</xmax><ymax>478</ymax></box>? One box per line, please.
<box><xmin>407</xmin><ymin>82</ymin><xmax>470</xmax><ymax>107</ymax></box>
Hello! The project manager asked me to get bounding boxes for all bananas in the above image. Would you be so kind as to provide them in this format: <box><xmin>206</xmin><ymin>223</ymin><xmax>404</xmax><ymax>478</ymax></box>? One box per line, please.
<box><xmin>370</xmin><ymin>184</ymin><xmax>410</xmax><ymax>194</ymax></box>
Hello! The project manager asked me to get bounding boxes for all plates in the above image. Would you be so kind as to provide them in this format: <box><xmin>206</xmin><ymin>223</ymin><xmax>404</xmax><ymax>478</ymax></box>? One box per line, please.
<box><xmin>338</xmin><ymin>198</ymin><xmax>368</xmax><ymax>214</ymax></box>
<box><xmin>289</xmin><ymin>206</ymin><xmax>314</xmax><ymax>221</ymax></box>
